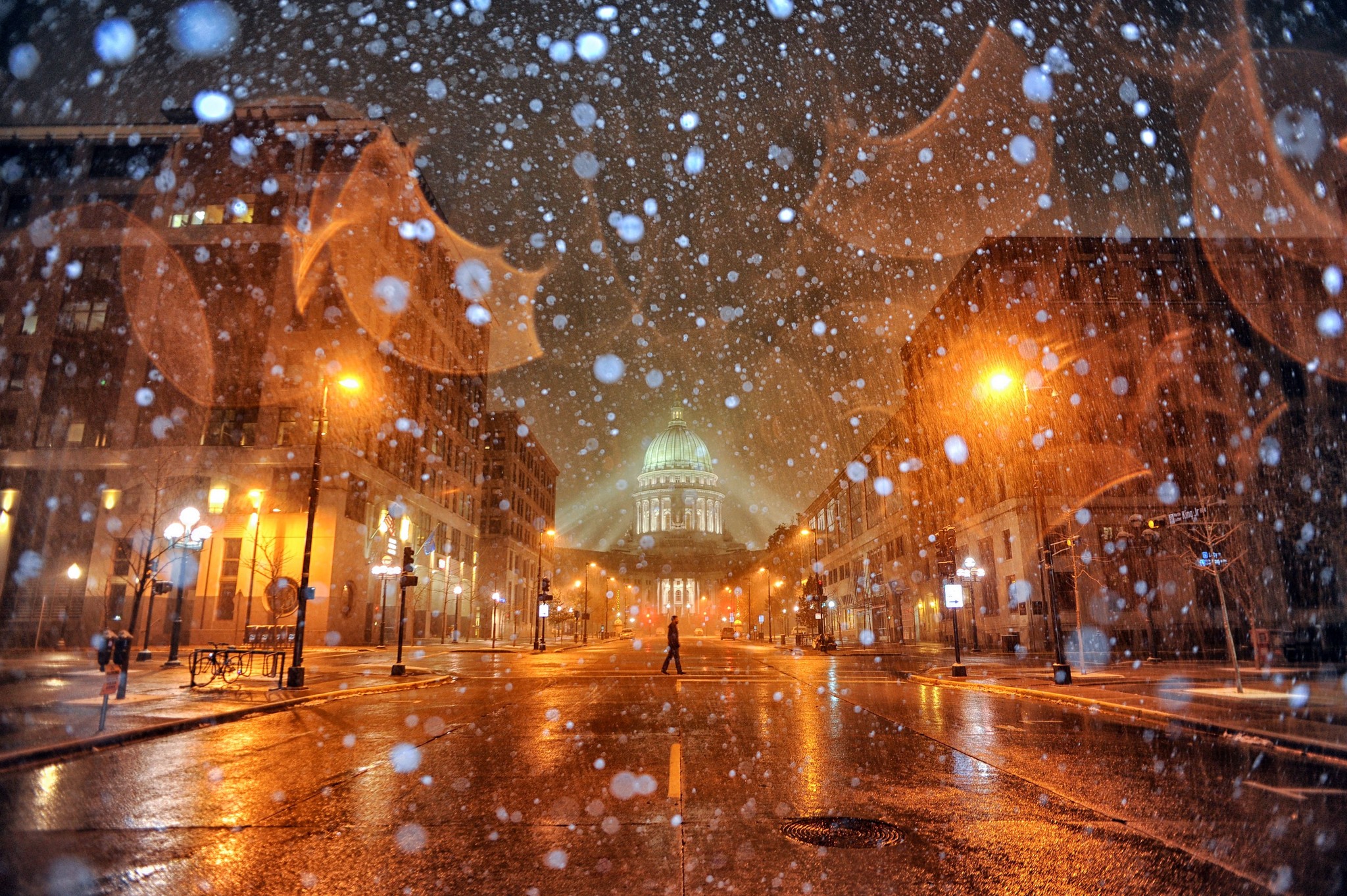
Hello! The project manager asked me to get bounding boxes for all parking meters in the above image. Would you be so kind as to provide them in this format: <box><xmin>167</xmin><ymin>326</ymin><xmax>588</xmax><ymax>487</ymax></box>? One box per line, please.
<box><xmin>97</xmin><ymin>628</ymin><xmax>132</xmax><ymax>731</ymax></box>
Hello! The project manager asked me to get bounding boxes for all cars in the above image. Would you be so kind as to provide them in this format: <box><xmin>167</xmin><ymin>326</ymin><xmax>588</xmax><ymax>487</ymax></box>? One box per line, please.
<box><xmin>693</xmin><ymin>628</ymin><xmax>703</xmax><ymax>636</ymax></box>
<box><xmin>619</xmin><ymin>628</ymin><xmax>635</xmax><ymax>640</ymax></box>
<box><xmin>720</xmin><ymin>627</ymin><xmax>736</xmax><ymax>641</ymax></box>
<box><xmin>655</xmin><ymin>627</ymin><xmax>665</xmax><ymax>636</ymax></box>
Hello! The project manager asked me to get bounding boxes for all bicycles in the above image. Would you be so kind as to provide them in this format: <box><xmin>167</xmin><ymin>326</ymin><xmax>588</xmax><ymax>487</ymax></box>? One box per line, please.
<box><xmin>799</xmin><ymin>633</ymin><xmax>807</xmax><ymax>646</ymax></box>
<box><xmin>193</xmin><ymin>641</ymin><xmax>243</xmax><ymax>688</ymax></box>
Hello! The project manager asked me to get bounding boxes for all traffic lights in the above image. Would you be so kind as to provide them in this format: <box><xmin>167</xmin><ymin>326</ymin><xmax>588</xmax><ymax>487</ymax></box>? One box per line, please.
<box><xmin>934</xmin><ymin>526</ymin><xmax>958</xmax><ymax>557</ymax></box>
<box><xmin>818</xmin><ymin>581</ymin><xmax>822</xmax><ymax>594</ymax></box>
<box><xmin>403</xmin><ymin>547</ymin><xmax>414</xmax><ymax>572</ymax></box>
<box><xmin>401</xmin><ymin>574</ymin><xmax>417</xmax><ymax>587</ymax></box>
<box><xmin>1147</xmin><ymin>518</ymin><xmax>1165</xmax><ymax>528</ymax></box>
<box><xmin>937</xmin><ymin>561</ymin><xmax>954</xmax><ymax>576</ymax></box>
<box><xmin>155</xmin><ymin>581</ymin><xmax>172</xmax><ymax>593</ymax></box>
<box><xmin>542</xmin><ymin>577</ymin><xmax>550</xmax><ymax>591</ymax></box>
<box><xmin>1066</xmin><ymin>536</ymin><xmax>1081</xmax><ymax>547</ymax></box>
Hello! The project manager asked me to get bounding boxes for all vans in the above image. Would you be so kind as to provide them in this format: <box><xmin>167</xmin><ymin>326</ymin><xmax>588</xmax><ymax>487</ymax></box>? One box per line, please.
<box><xmin>791</xmin><ymin>627</ymin><xmax>806</xmax><ymax>634</ymax></box>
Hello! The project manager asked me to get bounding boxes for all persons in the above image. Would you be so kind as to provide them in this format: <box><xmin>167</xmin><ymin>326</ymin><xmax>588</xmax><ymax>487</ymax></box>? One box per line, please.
<box><xmin>661</xmin><ymin>616</ymin><xmax>687</xmax><ymax>675</ymax></box>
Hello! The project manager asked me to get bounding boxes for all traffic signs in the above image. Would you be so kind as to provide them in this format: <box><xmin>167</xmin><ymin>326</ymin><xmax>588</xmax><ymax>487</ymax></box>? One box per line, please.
<box><xmin>944</xmin><ymin>584</ymin><xmax>964</xmax><ymax>608</ymax></box>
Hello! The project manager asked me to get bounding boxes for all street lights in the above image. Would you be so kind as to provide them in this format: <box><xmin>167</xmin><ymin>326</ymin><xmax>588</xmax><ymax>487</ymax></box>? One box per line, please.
<box><xmin>534</xmin><ymin>528</ymin><xmax>554</xmax><ymax>649</ymax></box>
<box><xmin>624</xmin><ymin>585</ymin><xmax>631</xmax><ymax>628</ymax></box>
<box><xmin>986</xmin><ymin>371</ymin><xmax>1073</xmax><ymax>686</ymax></box>
<box><xmin>702</xmin><ymin>596</ymin><xmax>708</xmax><ymax>636</ymax></box>
<box><xmin>286</xmin><ymin>375</ymin><xmax>360</xmax><ymax>688</ymax></box>
<box><xmin>164</xmin><ymin>506</ymin><xmax>213</xmax><ymax>667</ymax></box>
<box><xmin>740</xmin><ymin>577</ymin><xmax>751</xmax><ymax>640</ymax></box>
<box><xmin>605</xmin><ymin>577</ymin><xmax>614</xmax><ymax>639</ymax></box>
<box><xmin>802</xmin><ymin>529</ymin><xmax>821</xmax><ymax>649</ymax></box>
<box><xmin>760</xmin><ymin>567</ymin><xmax>772</xmax><ymax>643</ymax></box>
<box><xmin>583</xmin><ymin>562</ymin><xmax>595</xmax><ymax>644</ymax></box>
<box><xmin>1116</xmin><ymin>515</ymin><xmax>1162</xmax><ymax>663</ymax></box>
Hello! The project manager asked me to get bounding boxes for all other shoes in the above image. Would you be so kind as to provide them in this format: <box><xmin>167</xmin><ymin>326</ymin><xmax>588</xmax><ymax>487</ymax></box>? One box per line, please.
<box><xmin>677</xmin><ymin>671</ymin><xmax>686</xmax><ymax>674</ymax></box>
<box><xmin>661</xmin><ymin>670</ymin><xmax>670</xmax><ymax>675</ymax></box>
